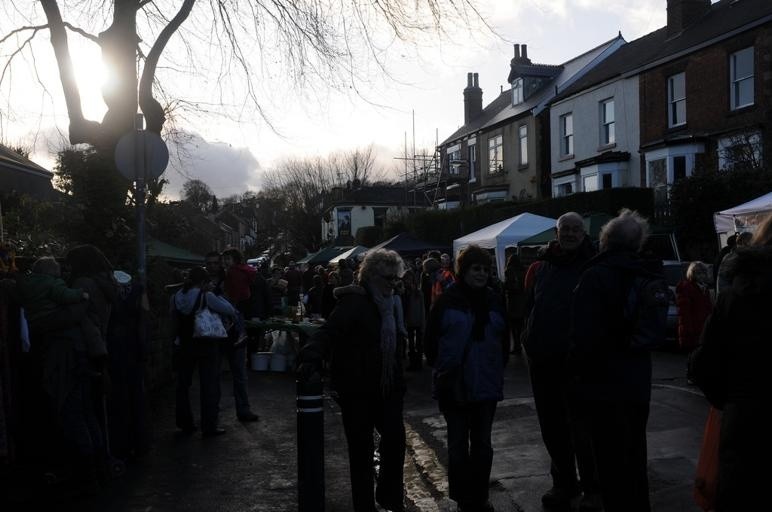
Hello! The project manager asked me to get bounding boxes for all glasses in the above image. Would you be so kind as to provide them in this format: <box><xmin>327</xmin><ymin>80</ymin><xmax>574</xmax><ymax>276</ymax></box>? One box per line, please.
<box><xmin>379</xmin><ymin>272</ymin><xmax>401</xmax><ymax>281</ymax></box>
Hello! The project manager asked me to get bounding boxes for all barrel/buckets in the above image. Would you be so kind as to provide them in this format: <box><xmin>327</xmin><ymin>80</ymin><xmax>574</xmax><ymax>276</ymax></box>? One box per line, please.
<box><xmin>251</xmin><ymin>351</ymin><xmax>272</xmax><ymax>371</ymax></box>
<box><xmin>269</xmin><ymin>351</ymin><xmax>287</xmax><ymax>372</ymax></box>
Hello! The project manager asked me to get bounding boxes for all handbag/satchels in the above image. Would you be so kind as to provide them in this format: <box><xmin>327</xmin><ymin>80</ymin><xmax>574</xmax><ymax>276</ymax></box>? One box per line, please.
<box><xmin>190</xmin><ymin>309</ymin><xmax>228</xmax><ymax>339</ymax></box>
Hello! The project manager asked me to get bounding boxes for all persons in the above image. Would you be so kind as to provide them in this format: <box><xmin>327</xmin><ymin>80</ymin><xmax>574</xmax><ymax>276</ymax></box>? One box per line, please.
<box><xmin>0</xmin><ymin>208</ymin><xmax>772</xmax><ymax>512</ymax></box>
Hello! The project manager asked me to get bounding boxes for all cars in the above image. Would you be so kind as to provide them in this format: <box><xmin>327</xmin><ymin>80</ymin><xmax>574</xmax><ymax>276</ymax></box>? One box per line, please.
<box><xmin>660</xmin><ymin>259</ymin><xmax>716</xmax><ymax>352</ymax></box>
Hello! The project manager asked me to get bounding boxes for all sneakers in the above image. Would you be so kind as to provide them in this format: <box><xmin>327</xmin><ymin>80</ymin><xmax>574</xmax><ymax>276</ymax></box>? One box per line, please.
<box><xmin>249</xmin><ymin>414</ymin><xmax>258</xmax><ymax>422</ymax></box>
<box><xmin>542</xmin><ymin>486</ymin><xmax>566</xmax><ymax>507</ymax></box>
<box><xmin>216</xmin><ymin>428</ymin><xmax>226</xmax><ymax>435</ymax></box>
